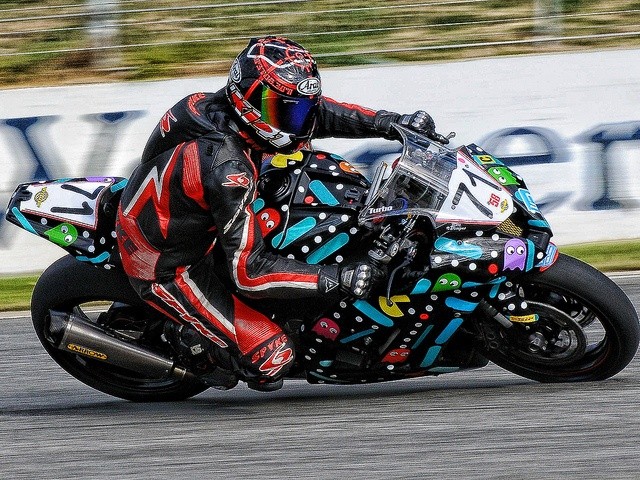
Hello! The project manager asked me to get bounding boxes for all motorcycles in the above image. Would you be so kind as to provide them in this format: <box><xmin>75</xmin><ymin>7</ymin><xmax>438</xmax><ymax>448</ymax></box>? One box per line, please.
<box><xmin>6</xmin><ymin>121</ymin><xmax>640</xmax><ymax>403</ymax></box>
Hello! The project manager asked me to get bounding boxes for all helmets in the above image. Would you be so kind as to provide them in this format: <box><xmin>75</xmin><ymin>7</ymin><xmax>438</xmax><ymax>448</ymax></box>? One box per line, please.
<box><xmin>225</xmin><ymin>35</ymin><xmax>322</xmax><ymax>155</ymax></box>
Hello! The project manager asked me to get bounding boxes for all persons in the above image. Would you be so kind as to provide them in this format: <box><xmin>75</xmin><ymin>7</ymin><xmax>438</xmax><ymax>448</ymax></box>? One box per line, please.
<box><xmin>114</xmin><ymin>37</ymin><xmax>449</xmax><ymax>389</ymax></box>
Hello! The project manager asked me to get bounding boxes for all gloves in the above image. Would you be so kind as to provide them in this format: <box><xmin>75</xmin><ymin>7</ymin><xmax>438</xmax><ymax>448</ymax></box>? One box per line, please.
<box><xmin>318</xmin><ymin>261</ymin><xmax>385</xmax><ymax>301</ymax></box>
<box><xmin>373</xmin><ymin>110</ymin><xmax>450</xmax><ymax>149</ymax></box>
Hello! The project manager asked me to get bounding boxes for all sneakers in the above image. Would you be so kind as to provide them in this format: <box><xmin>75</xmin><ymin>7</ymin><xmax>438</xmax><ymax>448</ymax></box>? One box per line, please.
<box><xmin>163</xmin><ymin>320</ymin><xmax>216</xmax><ymax>375</ymax></box>
